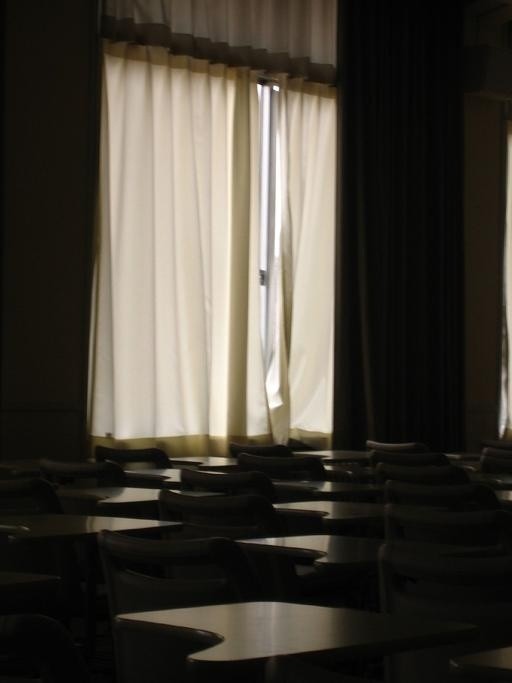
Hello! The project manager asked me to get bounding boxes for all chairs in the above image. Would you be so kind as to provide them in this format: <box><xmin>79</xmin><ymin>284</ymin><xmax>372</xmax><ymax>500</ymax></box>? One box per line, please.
<box><xmin>1</xmin><ymin>427</ymin><xmax>512</xmax><ymax>683</ymax></box>
